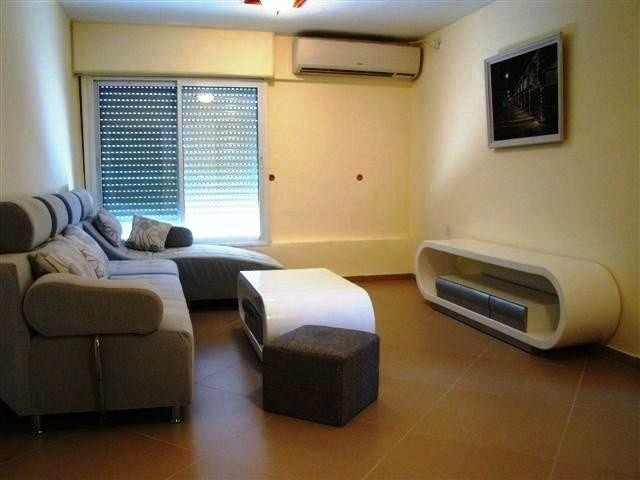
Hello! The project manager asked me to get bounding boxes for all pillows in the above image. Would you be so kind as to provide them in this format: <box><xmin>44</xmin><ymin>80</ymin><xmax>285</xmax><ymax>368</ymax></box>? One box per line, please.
<box><xmin>29</xmin><ymin>206</ymin><xmax>173</xmax><ymax>282</ymax></box>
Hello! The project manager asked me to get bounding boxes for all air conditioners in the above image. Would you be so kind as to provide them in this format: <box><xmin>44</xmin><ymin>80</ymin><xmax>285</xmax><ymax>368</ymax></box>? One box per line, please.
<box><xmin>292</xmin><ymin>36</ymin><xmax>423</xmax><ymax>82</ymax></box>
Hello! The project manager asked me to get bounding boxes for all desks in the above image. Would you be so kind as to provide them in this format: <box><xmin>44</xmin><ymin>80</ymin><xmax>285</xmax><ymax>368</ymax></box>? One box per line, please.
<box><xmin>415</xmin><ymin>238</ymin><xmax>620</xmax><ymax>353</ymax></box>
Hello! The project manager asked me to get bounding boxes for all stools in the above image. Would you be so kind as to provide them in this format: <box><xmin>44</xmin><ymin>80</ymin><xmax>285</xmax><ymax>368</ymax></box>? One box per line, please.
<box><xmin>262</xmin><ymin>323</ymin><xmax>381</xmax><ymax>429</ymax></box>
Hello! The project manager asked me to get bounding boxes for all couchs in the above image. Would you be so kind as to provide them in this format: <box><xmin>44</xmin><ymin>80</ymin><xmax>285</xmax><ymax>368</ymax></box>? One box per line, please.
<box><xmin>0</xmin><ymin>188</ymin><xmax>284</xmax><ymax>439</ymax></box>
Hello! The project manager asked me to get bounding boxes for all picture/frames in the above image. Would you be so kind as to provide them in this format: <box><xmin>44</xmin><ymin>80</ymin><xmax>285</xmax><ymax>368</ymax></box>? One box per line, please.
<box><xmin>483</xmin><ymin>31</ymin><xmax>566</xmax><ymax>147</ymax></box>
